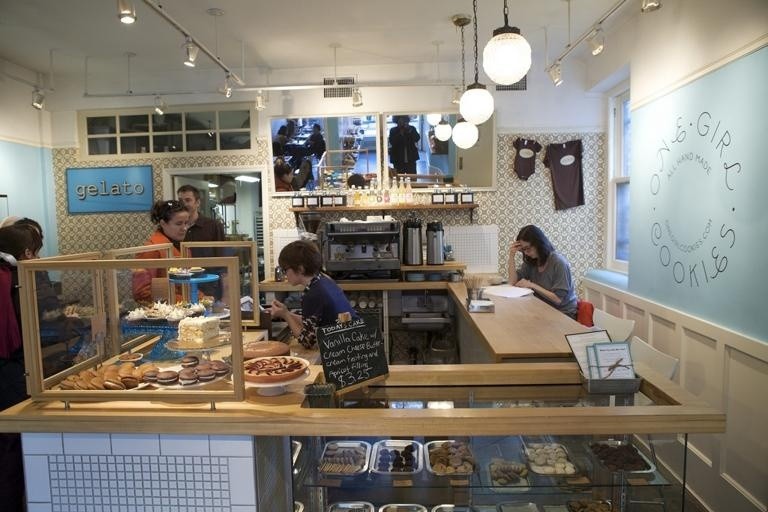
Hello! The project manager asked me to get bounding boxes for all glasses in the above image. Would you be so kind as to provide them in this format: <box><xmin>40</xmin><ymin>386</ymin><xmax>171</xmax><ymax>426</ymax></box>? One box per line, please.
<box><xmin>282</xmin><ymin>266</ymin><xmax>292</xmax><ymax>275</ymax></box>
<box><xmin>518</xmin><ymin>245</ymin><xmax>532</xmax><ymax>253</ymax></box>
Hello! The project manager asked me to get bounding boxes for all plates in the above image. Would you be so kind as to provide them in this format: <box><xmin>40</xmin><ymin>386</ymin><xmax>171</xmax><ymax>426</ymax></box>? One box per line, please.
<box><xmin>407</xmin><ymin>272</ymin><xmax>426</xmax><ymax>283</ymax></box>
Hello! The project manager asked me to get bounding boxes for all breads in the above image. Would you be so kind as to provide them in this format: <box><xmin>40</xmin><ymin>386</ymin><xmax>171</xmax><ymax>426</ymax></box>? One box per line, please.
<box><xmin>525</xmin><ymin>443</ymin><xmax>575</xmax><ymax>475</ymax></box>
<box><xmin>491</xmin><ymin>456</ymin><xmax>529</xmax><ymax>485</ymax></box>
<box><xmin>378</xmin><ymin>444</ymin><xmax>418</xmax><ymax>472</ymax></box>
<box><xmin>592</xmin><ymin>443</ymin><xmax>647</xmax><ymax>474</ymax></box>
<box><xmin>567</xmin><ymin>498</ymin><xmax>613</xmax><ymax>512</ymax></box>
<box><xmin>429</xmin><ymin>441</ymin><xmax>477</xmax><ymax>474</ymax></box>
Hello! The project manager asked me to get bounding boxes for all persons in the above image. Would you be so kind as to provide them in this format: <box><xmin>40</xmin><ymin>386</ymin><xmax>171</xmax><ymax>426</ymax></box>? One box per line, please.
<box><xmin>304</xmin><ymin>125</ymin><xmax>325</xmax><ymax>155</ymax></box>
<box><xmin>12</xmin><ymin>216</ymin><xmax>68</xmax><ymax>346</ymax></box>
<box><xmin>177</xmin><ymin>185</ymin><xmax>228</xmax><ymax>293</ymax></box>
<box><xmin>385</xmin><ymin>113</ymin><xmax>425</xmax><ymax>179</ymax></box>
<box><xmin>507</xmin><ymin>224</ymin><xmax>579</xmax><ymax>321</ymax></box>
<box><xmin>270</xmin><ymin>240</ymin><xmax>361</xmax><ymax>351</ymax></box>
<box><xmin>275</xmin><ymin>159</ymin><xmax>296</xmax><ymax>191</ymax></box>
<box><xmin>274</xmin><ymin>124</ymin><xmax>291</xmax><ymax>148</ymax></box>
<box><xmin>0</xmin><ymin>225</ymin><xmax>41</xmax><ymax>413</ymax></box>
<box><xmin>129</xmin><ymin>199</ymin><xmax>205</xmax><ymax>304</ymax></box>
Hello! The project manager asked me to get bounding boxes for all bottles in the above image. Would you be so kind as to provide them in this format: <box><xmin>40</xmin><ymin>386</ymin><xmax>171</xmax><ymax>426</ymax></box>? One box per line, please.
<box><xmin>348</xmin><ymin>294</ymin><xmax>382</xmax><ymax>309</ymax></box>
<box><xmin>347</xmin><ymin>175</ymin><xmax>415</xmax><ymax>206</ymax></box>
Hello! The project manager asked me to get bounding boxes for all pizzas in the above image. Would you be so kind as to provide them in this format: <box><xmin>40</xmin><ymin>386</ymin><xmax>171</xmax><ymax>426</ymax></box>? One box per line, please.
<box><xmin>243</xmin><ymin>355</ymin><xmax>310</xmax><ymax>383</ymax></box>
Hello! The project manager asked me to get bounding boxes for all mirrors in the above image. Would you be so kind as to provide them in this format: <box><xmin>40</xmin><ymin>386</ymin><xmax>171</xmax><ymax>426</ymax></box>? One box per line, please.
<box><xmin>267</xmin><ymin>113</ymin><xmax>382</xmax><ymax>192</ymax></box>
<box><xmin>384</xmin><ymin>112</ymin><xmax>497</xmax><ymax>191</ymax></box>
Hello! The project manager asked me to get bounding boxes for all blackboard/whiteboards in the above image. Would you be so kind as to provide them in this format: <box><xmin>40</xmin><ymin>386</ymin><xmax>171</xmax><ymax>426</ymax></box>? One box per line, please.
<box><xmin>317</xmin><ymin>314</ymin><xmax>389</xmax><ymax>391</ymax></box>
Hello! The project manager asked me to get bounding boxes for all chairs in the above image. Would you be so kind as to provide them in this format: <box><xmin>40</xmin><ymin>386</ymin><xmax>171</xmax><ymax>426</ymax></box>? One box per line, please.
<box><xmin>590</xmin><ymin>306</ymin><xmax>635</xmax><ymax>347</ymax></box>
<box><xmin>626</xmin><ymin>332</ymin><xmax>680</xmax><ymax>381</ymax></box>
<box><xmin>575</xmin><ymin>299</ymin><xmax>594</xmax><ymax>330</ymax></box>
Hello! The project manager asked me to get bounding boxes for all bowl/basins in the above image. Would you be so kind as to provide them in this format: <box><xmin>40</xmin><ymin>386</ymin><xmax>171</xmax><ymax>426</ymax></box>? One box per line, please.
<box><xmin>449</xmin><ymin>271</ymin><xmax>461</xmax><ymax>281</ymax></box>
<box><xmin>429</xmin><ymin>273</ymin><xmax>441</xmax><ymax>281</ymax></box>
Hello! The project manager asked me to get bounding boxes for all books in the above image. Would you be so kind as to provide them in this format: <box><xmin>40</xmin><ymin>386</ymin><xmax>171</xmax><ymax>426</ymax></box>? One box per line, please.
<box><xmin>480</xmin><ymin>283</ymin><xmax>535</xmax><ymax>299</ymax></box>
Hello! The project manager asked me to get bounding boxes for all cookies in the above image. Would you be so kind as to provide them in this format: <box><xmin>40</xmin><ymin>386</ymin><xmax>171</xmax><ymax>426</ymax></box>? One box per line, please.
<box><xmin>320</xmin><ymin>442</ymin><xmax>367</xmax><ymax>473</ymax></box>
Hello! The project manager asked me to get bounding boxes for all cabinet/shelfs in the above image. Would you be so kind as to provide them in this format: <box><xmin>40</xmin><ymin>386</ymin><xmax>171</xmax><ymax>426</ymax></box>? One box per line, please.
<box><xmin>289</xmin><ymin>362</ymin><xmax>727</xmax><ymax>511</ymax></box>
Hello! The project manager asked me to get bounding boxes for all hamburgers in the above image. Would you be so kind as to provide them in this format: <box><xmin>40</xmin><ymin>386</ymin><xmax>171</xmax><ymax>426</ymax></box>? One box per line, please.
<box><xmin>59</xmin><ymin>356</ymin><xmax>230</xmax><ymax>390</ymax></box>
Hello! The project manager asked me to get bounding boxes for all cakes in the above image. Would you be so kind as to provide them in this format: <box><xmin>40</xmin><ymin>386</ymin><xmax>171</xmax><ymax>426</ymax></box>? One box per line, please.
<box><xmin>177</xmin><ymin>315</ymin><xmax>220</xmax><ymax>344</ymax></box>
<box><xmin>168</xmin><ymin>266</ymin><xmax>207</xmax><ymax>280</ymax></box>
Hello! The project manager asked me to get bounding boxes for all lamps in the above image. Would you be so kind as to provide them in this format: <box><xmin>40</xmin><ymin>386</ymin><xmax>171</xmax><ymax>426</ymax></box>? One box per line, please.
<box><xmin>78</xmin><ymin>42</ymin><xmax>471</xmax><ymax>114</ymax></box>
<box><xmin>30</xmin><ymin>84</ymin><xmax>45</xmax><ymax>110</ymax></box>
<box><xmin>482</xmin><ymin>1</ymin><xmax>533</xmax><ymax>85</ymax></box>
<box><xmin>427</xmin><ymin>113</ymin><xmax>452</xmax><ymax>144</ymax></box>
<box><xmin>450</xmin><ymin>0</ymin><xmax>495</xmax><ymax>150</ymax></box>
<box><xmin>115</xmin><ymin>1</ymin><xmax>246</xmax><ymax>99</ymax></box>
<box><xmin>543</xmin><ymin>0</ymin><xmax>669</xmax><ymax>88</ymax></box>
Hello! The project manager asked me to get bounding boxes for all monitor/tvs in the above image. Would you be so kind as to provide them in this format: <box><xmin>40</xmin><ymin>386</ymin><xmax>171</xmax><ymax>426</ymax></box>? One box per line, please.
<box><xmin>240</xmin><ymin>295</ymin><xmax>264</xmax><ymax>311</ymax></box>
